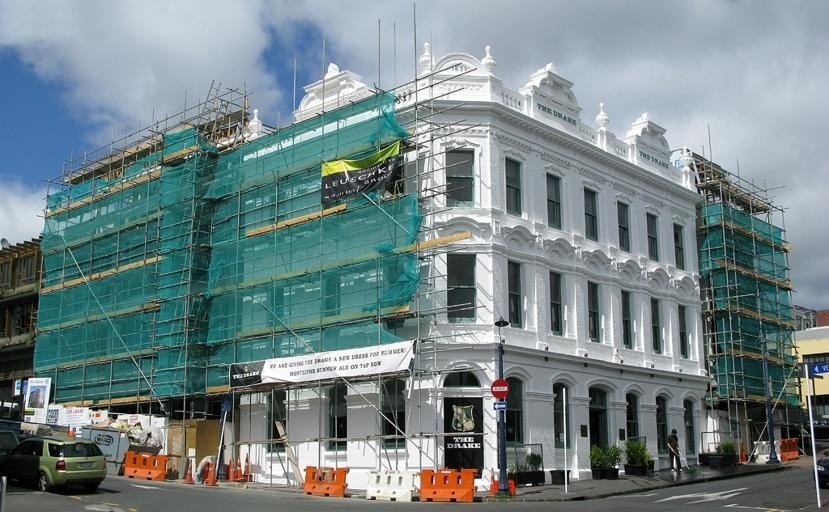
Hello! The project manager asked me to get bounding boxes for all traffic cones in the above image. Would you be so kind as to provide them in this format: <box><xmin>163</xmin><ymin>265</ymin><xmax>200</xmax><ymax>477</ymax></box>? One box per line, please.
<box><xmin>228</xmin><ymin>458</ymin><xmax>249</xmax><ymax>483</ymax></box>
<box><xmin>203</xmin><ymin>460</ymin><xmax>218</xmax><ymax>486</ymax></box>
<box><xmin>183</xmin><ymin>459</ymin><xmax>194</xmax><ymax>484</ymax></box>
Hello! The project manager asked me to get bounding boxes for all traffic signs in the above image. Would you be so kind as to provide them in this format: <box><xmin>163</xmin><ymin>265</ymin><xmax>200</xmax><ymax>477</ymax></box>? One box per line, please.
<box><xmin>811</xmin><ymin>363</ymin><xmax>829</xmax><ymax>374</ymax></box>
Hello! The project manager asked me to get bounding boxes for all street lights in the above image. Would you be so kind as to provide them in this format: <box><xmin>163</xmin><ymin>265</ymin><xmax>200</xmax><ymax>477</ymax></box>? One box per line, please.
<box><xmin>494</xmin><ymin>315</ymin><xmax>510</xmax><ymax>491</ymax></box>
<box><xmin>760</xmin><ymin>337</ymin><xmax>780</xmax><ymax>464</ymax></box>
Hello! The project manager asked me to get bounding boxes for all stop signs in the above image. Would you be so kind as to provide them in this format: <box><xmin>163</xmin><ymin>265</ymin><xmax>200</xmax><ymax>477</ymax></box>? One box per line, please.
<box><xmin>492</xmin><ymin>380</ymin><xmax>510</xmax><ymax>398</ymax></box>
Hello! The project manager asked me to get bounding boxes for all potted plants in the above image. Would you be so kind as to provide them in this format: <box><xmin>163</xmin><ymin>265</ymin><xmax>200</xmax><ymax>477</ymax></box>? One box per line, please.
<box><xmin>508</xmin><ymin>452</ymin><xmax>544</xmax><ymax>485</ymax></box>
<box><xmin>591</xmin><ymin>440</ymin><xmax>655</xmax><ymax>480</ymax></box>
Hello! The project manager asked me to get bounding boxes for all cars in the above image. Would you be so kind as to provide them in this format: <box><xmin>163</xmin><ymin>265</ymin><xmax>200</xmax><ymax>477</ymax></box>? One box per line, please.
<box><xmin>814</xmin><ymin>450</ymin><xmax>829</xmax><ymax>488</ymax></box>
<box><xmin>1</xmin><ymin>429</ymin><xmax>107</xmax><ymax>493</ymax></box>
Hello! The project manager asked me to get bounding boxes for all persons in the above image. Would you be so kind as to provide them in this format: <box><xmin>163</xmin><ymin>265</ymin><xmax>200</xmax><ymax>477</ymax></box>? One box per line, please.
<box><xmin>667</xmin><ymin>428</ymin><xmax>683</xmax><ymax>471</ymax></box>
